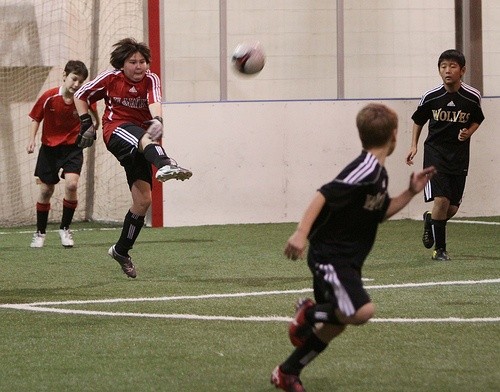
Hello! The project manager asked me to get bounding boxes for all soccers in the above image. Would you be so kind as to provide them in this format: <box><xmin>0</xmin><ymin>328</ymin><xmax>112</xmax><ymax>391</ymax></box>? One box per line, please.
<box><xmin>231</xmin><ymin>42</ymin><xmax>265</xmax><ymax>75</ymax></box>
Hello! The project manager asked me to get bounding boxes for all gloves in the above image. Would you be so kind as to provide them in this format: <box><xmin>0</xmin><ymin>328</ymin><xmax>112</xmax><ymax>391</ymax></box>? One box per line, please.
<box><xmin>142</xmin><ymin>116</ymin><xmax>163</xmax><ymax>142</ymax></box>
<box><xmin>75</xmin><ymin>113</ymin><xmax>98</xmax><ymax>149</ymax></box>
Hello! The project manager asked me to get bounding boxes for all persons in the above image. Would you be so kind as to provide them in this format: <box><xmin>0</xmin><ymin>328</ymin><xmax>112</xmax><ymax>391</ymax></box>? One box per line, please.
<box><xmin>25</xmin><ymin>60</ymin><xmax>97</xmax><ymax>249</ymax></box>
<box><xmin>73</xmin><ymin>37</ymin><xmax>192</xmax><ymax>278</ymax></box>
<box><xmin>405</xmin><ymin>49</ymin><xmax>486</xmax><ymax>261</ymax></box>
<box><xmin>270</xmin><ymin>102</ymin><xmax>437</xmax><ymax>392</ymax></box>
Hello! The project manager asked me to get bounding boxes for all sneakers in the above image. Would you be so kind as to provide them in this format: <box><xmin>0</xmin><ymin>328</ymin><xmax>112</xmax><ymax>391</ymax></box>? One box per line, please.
<box><xmin>271</xmin><ymin>364</ymin><xmax>305</xmax><ymax>392</ymax></box>
<box><xmin>432</xmin><ymin>248</ymin><xmax>451</xmax><ymax>261</ymax></box>
<box><xmin>108</xmin><ymin>244</ymin><xmax>137</xmax><ymax>278</ymax></box>
<box><xmin>30</xmin><ymin>230</ymin><xmax>46</xmax><ymax>248</ymax></box>
<box><xmin>289</xmin><ymin>297</ymin><xmax>315</xmax><ymax>346</ymax></box>
<box><xmin>423</xmin><ymin>211</ymin><xmax>434</xmax><ymax>249</ymax></box>
<box><xmin>59</xmin><ymin>228</ymin><xmax>74</xmax><ymax>247</ymax></box>
<box><xmin>155</xmin><ymin>163</ymin><xmax>193</xmax><ymax>183</ymax></box>
